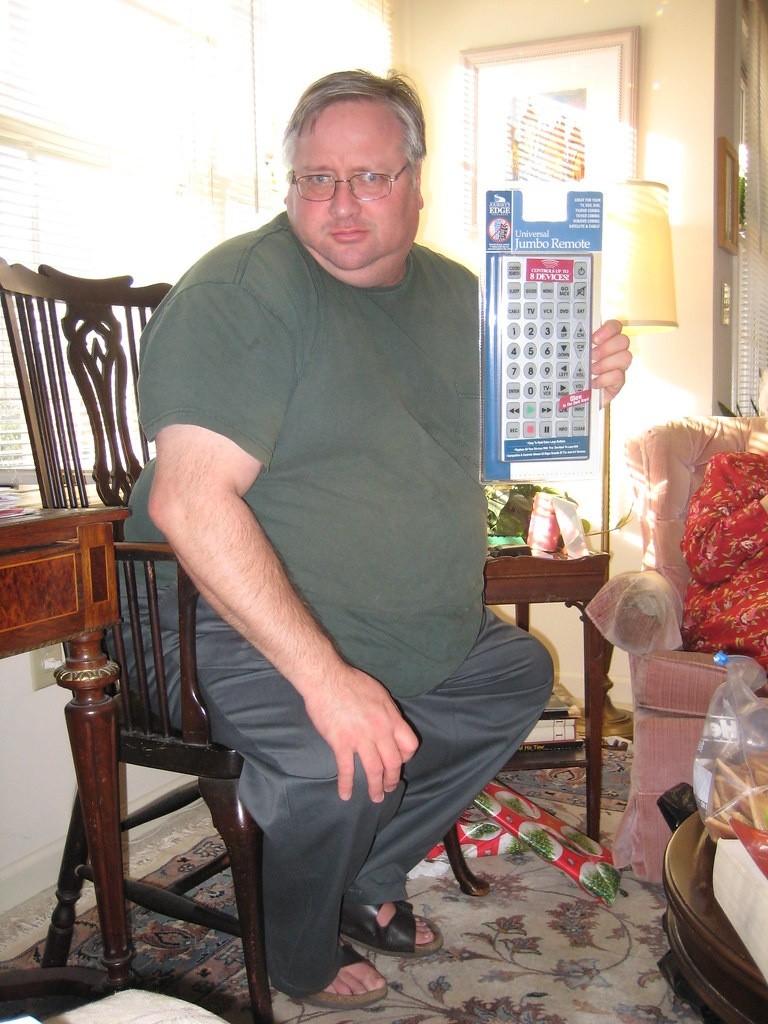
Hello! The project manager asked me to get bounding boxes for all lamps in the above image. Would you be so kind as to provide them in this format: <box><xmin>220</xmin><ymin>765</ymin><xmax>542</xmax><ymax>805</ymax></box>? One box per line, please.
<box><xmin>576</xmin><ymin>177</ymin><xmax>678</xmax><ymax>741</ymax></box>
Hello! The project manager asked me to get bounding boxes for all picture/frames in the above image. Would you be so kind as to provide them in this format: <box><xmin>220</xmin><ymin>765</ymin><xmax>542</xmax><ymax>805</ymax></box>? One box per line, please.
<box><xmin>717</xmin><ymin>136</ymin><xmax>740</xmax><ymax>255</ymax></box>
<box><xmin>451</xmin><ymin>23</ymin><xmax>643</xmax><ymax>256</ymax></box>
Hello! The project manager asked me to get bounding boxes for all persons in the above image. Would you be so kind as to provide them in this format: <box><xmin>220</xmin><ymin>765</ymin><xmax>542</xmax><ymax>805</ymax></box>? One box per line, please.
<box><xmin>106</xmin><ymin>72</ymin><xmax>632</xmax><ymax>1009</ymax></box>
<box><xmin>683</xmin><ymin>371</ymin><xmax>767</xmax><ymax>664</ymax></box>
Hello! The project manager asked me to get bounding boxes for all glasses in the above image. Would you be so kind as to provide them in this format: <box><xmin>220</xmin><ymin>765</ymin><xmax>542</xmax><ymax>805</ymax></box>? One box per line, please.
<box><xmin>292</xmin><ymin>161</ymin><xmax>411</xmax><ymax>202</ymax></box>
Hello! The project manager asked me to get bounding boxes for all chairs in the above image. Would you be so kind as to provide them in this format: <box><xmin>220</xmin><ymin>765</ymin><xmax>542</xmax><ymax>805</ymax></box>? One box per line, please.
<box><xmin>0</xmin><ymin>257</ymin><xmax>487</xmax><ymax>1024</ymax></box>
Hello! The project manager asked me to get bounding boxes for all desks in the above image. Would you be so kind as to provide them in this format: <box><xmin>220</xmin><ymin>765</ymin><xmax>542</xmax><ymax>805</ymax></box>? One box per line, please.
<box><xmin>482</xmin><ymin>549</ymin><xmax>613</xmax><ymax>843</ymax></box>
<box><xmin>654</xmin><ymin>784</ymin><xmax>768</xmax><ymax>1024</ymax></box>
<box><xmin>0</xmin><ymin>506</ymin><xmax>135</xmax><ymax>996</ymax></box>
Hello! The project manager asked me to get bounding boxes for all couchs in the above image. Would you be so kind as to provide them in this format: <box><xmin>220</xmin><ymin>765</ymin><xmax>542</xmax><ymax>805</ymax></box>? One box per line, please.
<box><xmin>581</xmin><ymin>416</ymin><xmax>768</xmax><ymax>882</ymax></box>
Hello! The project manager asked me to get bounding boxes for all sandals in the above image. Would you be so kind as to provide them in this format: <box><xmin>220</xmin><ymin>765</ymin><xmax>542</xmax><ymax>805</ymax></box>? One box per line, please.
<box><xmin>339</xmin><ymin>900</ymin><xmax>443</xmax><ymax>957</ymax></box>
<box><xmin>298</xmin><ymin>936</ymin><xmax>388</xmax><ymax>1009</ymax></box>
